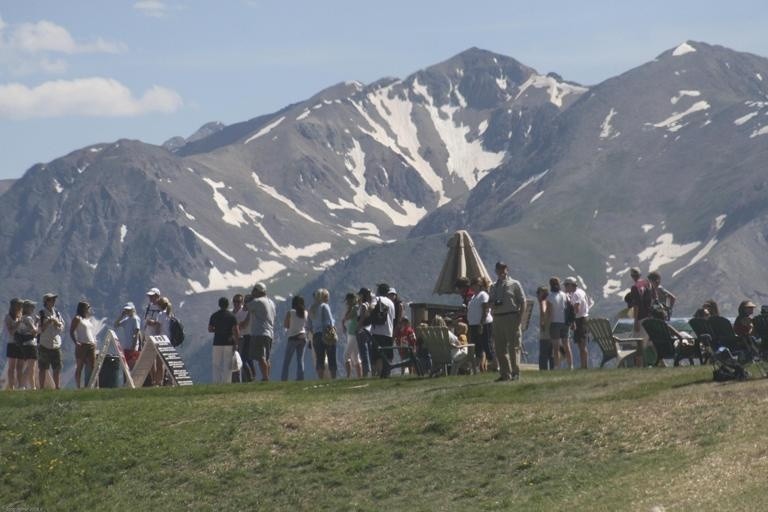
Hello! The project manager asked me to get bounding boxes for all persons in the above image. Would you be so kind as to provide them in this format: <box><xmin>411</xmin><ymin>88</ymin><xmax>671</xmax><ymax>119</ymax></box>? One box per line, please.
<box><xmin>208</xmin><ymin>282</ymin><xmax>276</xmax><ymax>383</ymax></box>
<box><xmin>536</xmin><ymin>276</ymin><xmax>590</xmax><ymax>369</ymax></box>
<box><xmin>116</xmin><ymin>288</ymin><xmax>173</xmax><ymax>385</ymax></box>
<box><xmin>488</xmin><ymin>261</ymin><xmax>526</xmax><ymax>381</ymax></box>
<box><xmin>654</xmin><ymin>298</ymin><xmax>766</xmax><ymax>359</ymax></box>
<box><xmin>397</xmin><ymin>314</ymin><xmax>471</xmax><ymax>376</ymax></box>
<box><xmin>618</xmin><ymin>267</ymin><xmax>675</xmax><ymax>367</ymax></box>
<box><xmin>282</xmin><ymin>296</ymin><xmax>308</xmax><ymax>381</ymax></box>
<box><xmin>457</xmin><ymin>277</ymin><xmax>499</xmax><ymax>371</ymax></box>
<box><xmin>70</xmin><ymin>302</ymin><xmax>98</xmax><ymax>390</ymax></box>
<box><xmin>342</xmin><ymin>284</ymin><xmax>403</xmax><ymax>378</ymax></box>
<box><xmin>4</xmin><ymin>287</ymin><xmax>65</xmax><ymax>393</ymax></box>
<box><xmin>305</xmin><ymin>288</ymin><xmax>337</xmax><ymax>379</ymax></box>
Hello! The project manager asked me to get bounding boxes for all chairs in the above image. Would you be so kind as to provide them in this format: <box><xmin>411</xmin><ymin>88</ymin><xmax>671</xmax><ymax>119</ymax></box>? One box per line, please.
<box><xmin>415</xmin><ymin>326</ymin><xmax>477</xmax><ymax>379</ymax></box>
<box><xmin>583</xmin><ymin>318</ymin><xmax>645</xmax><ymax>369</ymax></box>
<box><xmin>640</xmin><ymin>317</ymin><xmax>768</xmax><ymax>380</ymax></box>
<box><xmin>362</xmin><ymin>327</ymin><xmax>425</xmax><ymax>378</ymax></box>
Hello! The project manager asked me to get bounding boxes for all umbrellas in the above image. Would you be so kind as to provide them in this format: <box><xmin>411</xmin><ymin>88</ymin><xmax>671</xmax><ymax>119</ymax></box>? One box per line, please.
<box><xmin>430</xmin><ymin>228</ymin><xmax>494</xmax><ymax>296</ymax></box>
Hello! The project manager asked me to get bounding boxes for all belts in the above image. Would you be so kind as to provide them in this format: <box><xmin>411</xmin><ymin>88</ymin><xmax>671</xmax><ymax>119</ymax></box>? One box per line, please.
<box><xmin>493</xmin><ymin>311</ymin><xmax>517</xmax><ymax>316</ymax></box>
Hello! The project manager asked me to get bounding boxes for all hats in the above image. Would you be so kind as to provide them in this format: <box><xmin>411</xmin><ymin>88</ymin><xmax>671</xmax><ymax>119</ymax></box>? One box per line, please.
<box><xmin>374</xmin><ymin>283</ymin><xmax>389</xmax><ymax>292</ymax></box>
<box><xmin>559</xmin><ymin>276</ymin><xmax>577</xmax><ymax>287</ymax></box>
<box><xmin>10</xmin><ymin>297</ymin><xmax>37</xmax><ymax>307</ymax></box>
<box><xmin>144</xmin><ymin>287</ymin><xmax>160</xmax><ymax>296</ymax></box>
<box><xmin>629</xmin><ymin>266</ymin><xmax>641</xmax><ymax>275</ymax></box>
<box><xmin>494</xmin><ymin>261</ymin><xmax>507</xmax><ymax>270</ymax></box>
<box><xmin>355</xmin><ymin>287</ymin><xmax>370</xmax><ymax>296</ymax></box>
<box><xmin>386</xmin><ymin>287</ymin><xmax>397</xmax><ymax>295</ymax></box>
<box><xmin>249</xmin><ymin>282</ymin><xmax>266</xmax><ymax>293</ymax></box>
<box><xmin>42</xmin><ymin>293</ymin><xmax>57</xmax><ymax>302</ymax></box>
<box><xmin>122</xmin><ymin>302</ymin><xmax>135</xmax><ymax>310</ymax></box>
<box><xmin>742</xmin><ymin>301</ymin><xmax>755</xmax><ymax>308</ymax></box>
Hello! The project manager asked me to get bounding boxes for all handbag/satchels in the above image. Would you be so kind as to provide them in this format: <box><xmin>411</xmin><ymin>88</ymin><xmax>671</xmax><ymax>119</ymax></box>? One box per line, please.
<box><xmin>366</xmin><ymin>297</ymin><xmax>389</xmax><ymax>325</ymax></box>
<box><xmin>563</xmin><ymin>297</ymin><xmax>576</xmax><ymax>326</ymax></box>
<box><xmin>321</xmin><ymin>326</ymin><xmax>338</xmax><ymax>345</ymax></box>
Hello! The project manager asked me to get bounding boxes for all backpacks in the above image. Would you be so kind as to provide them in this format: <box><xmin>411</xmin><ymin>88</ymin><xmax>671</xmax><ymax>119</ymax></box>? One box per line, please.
<box><xmin>168</xmin><ymin>318</ymin><xmax>184</xmax><ymax>348</ymax></box>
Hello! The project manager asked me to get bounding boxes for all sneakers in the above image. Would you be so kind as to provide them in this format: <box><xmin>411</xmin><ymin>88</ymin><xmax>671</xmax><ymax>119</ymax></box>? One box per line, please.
<box><xmin>492</xmin><ymin>373</ymin><xmax>511</xmax><ymax>383</ymax></box>
<box><xmin>510</xmin><ymin>373</ymin><xmax>520</xmax><ymax>382</ymax></box>
